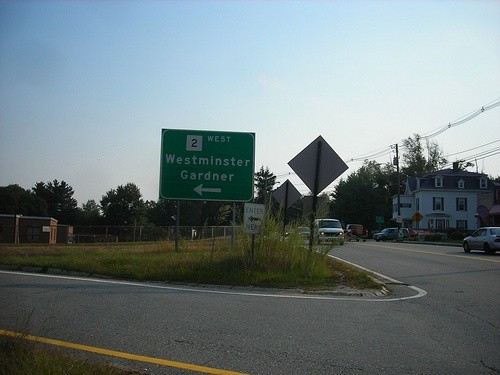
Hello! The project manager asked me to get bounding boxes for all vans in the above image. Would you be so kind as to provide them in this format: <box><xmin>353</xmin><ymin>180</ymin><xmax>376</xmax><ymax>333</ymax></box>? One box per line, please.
<box><xmin>314</xmin><ymin>219</ymin><xmax>344</xmax><ymax>245</ymax></box>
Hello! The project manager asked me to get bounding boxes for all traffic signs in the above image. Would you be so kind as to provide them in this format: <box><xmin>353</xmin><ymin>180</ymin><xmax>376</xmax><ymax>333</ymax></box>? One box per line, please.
<box><xmin>157</xmin><ymin>128</ymin><xmax>255</xmax><ymax>204</ymax></box>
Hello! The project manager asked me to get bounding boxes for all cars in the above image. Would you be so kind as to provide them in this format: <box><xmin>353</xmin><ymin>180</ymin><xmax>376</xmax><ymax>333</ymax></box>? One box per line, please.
<box><xmin>373</xmin><ymin>228</ymin><xmax>410</xmax><ymax>242</ymax></box>
<box><xmin>344</xmin><ymin>224</ymin><xmax>368</xmax><ymax>241</ymax></box>
<box><xmin>298</xmin><ymin>226</ymin><xmax>311</xmax><ymax>238</ymax></box>
<box><xmin>463</xmin><ymin>227</ymin><xmax>500</xmax><ymax>255</ymax></box>
<box><xmin>285</xmin><ymin>228</ymin><xmax>296</xmax><ymax>237</ymax></box>
